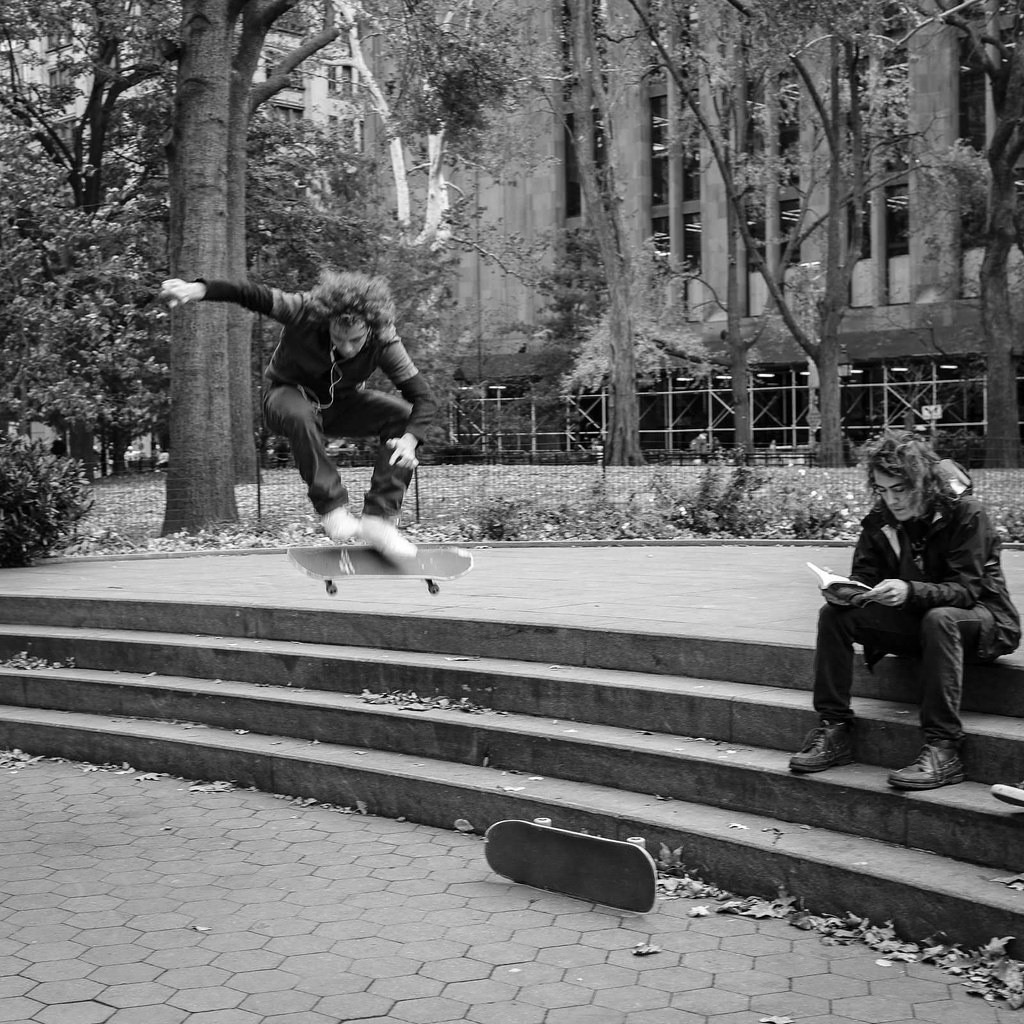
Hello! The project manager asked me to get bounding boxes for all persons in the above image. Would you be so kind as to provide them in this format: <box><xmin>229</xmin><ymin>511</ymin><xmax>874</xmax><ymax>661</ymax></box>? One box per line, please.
<box><xmin>690</xmin><ymin>432</ymin><xmax>720</xmax><ymax>454</ymax></box>
<box><xmin>264</xmin><ymin>439</ymin><xmax>292</xmax><ymax>469</ymax></box>
<box><xmin>788</xmin><ymin>427</ymin><xmax>1022</xmax><ymax>789</ymax></box>
<box><xmin>50</xmin><ymin>437</ymin><xmax>168</xmax><ymax>473</ymax></box>
<box><xmin>159</xmin><ymin>271</ymin><xmax>438</xmax><ymax>562</ymax></box>
<box><xmin>988</xmin><ymin>784</ymin><xmax>1024</xmax><ymax>806</ymax></box>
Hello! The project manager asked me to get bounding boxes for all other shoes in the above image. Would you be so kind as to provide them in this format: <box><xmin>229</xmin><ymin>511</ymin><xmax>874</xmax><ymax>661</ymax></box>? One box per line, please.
<box><xmin>321</xmin><ymin>506</ymin><xmax>359</xmax><ymax>543</ymax></box>
<box><xmin>359</xmin><ymin>515</ymin><xmax>418</xmax><ymax>561</ymax></box>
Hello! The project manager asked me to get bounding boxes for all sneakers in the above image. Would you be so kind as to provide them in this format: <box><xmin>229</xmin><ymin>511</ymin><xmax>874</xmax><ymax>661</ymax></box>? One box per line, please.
<box><xmin>789</xmin><ymin>720</ymin><xmax>851</xmax><ymax>771</ymax></box>
<box><xmin>990</xmin><ymin>780</ymin><xmax>1024</xmax><ymax>807</ymax></box>
<box><xmin>887</xmin><ymin>744</ymin><xmax>965</xmax><ymax>788</ymax></box>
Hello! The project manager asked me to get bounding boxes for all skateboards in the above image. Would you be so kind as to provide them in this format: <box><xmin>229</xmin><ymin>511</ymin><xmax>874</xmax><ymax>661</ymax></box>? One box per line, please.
<box><xmin>287</xmin><ymin>546</ymin><xmax>473</xmax><ymax>595</ymax></box>
<box><xmin>485</xmin><ymin>817</ymin><xmax>656</xmax><ymax>913</ymax></box>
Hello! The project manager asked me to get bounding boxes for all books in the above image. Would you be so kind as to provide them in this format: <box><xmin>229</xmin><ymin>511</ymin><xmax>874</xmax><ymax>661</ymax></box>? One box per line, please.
<box><xmin>806</xmin><ymin>562</ymin><xmax>875</xmax><ymax>608</ymax></box>
